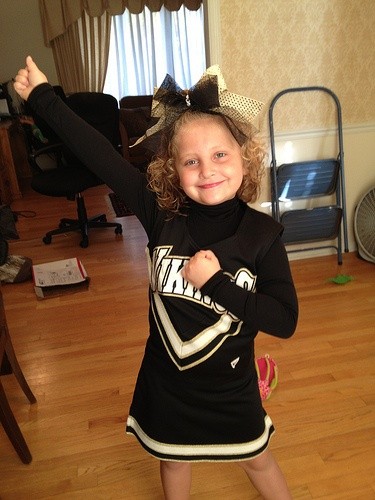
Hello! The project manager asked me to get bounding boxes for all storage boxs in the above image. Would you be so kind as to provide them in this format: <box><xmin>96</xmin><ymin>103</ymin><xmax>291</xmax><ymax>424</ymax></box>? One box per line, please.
<box><xmin>32</xmin><ymin>257</ymin><xmax>90</xmax><ymax>298</ymax></box>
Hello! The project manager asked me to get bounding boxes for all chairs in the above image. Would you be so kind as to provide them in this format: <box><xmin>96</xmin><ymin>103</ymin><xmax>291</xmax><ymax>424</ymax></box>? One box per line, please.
<box><xmin>266</xmin><ymin>86</ymin><xmax>349</xmax><ymax>266</ymax></box>
<box><xmin>0</xmin><ymin>290</ymin><xmax>37</xmax><ymax>464</ymax></box>
<box><xmin>27</xmin><ymin>92</ymin><xmax>123</xmax><ymax>248</ymax></box>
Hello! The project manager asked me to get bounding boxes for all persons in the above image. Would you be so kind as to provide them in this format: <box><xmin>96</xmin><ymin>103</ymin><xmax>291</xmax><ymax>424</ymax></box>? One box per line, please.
<box><xmin>14</xmin><ymin>55</ymin><xmax>299</xmax><ymax>500</ymax></box>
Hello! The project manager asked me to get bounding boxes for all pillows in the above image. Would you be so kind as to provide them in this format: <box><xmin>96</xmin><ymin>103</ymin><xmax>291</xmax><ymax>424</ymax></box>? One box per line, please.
<box><xmin>120</xmin><ymin>108</ymin><xmax>150</xmax><ymax>136</ymax></box>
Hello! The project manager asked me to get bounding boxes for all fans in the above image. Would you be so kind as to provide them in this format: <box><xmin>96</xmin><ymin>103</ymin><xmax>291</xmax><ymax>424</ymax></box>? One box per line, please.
<box><xmin>354</xmin><ymin>186</ymin><xmax>375</xmax><ymax>263</ymax></box>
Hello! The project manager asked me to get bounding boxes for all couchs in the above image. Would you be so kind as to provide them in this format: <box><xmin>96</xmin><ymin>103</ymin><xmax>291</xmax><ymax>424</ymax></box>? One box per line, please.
<box><xmin>120</xmin><ymin>96</ymin><xmax>159</xmax><ymax>159</ymax></box>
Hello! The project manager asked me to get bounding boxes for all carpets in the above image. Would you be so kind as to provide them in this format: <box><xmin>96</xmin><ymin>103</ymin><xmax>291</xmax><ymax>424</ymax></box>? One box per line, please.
<box><xmin>109</xmin><ymin>192</ymin><xmax>134</xmax><ymax>217</ymax></box>
